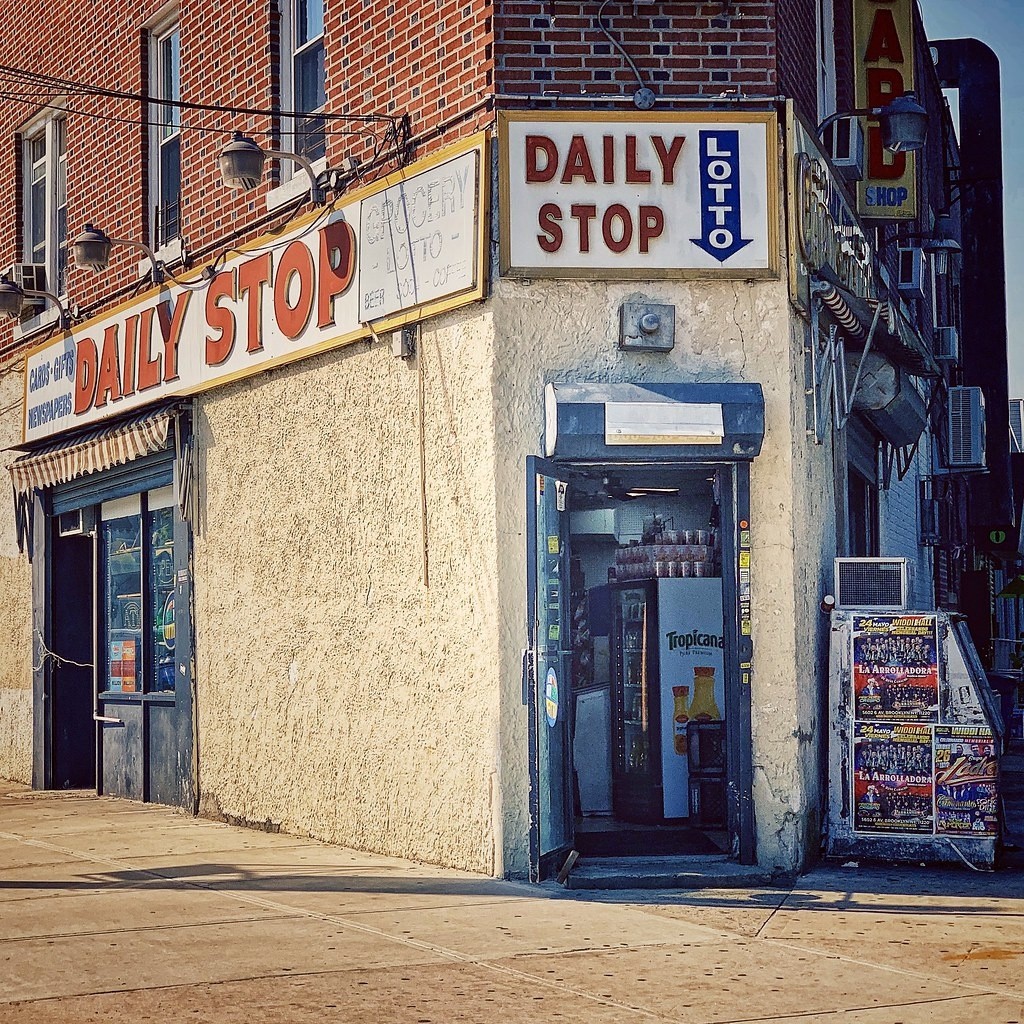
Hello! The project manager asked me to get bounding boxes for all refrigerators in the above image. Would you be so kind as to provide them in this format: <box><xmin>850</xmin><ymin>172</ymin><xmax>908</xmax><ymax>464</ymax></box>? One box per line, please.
<box><xmin>609</xmin><ymin>575</ymin><xmax>729</xmax><ymax>825</ymax></box>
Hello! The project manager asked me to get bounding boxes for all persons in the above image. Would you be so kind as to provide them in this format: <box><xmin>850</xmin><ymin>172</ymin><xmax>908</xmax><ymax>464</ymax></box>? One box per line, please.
<box><xmin>857</xmin><ymin>635</ymin><xmax>997</xmax><ymax>827</ymax></box>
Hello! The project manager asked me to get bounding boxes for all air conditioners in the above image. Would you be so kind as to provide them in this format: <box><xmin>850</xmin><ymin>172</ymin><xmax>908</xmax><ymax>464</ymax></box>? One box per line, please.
<box><xmin>936</xmin><ymin>325</ymin><xmax>958</xmax><ymax>363</ymax></box>
<box><xmin>898</xmin><ymin>247</ymin><xmax>926</xmax><ymax>297</ymax></box>
<box><xmin>1009</xmin><ymin>398</ymin><xmax>1023</xmax><ymax>453</ymax></box>
<box><xmin>919</xmin><ymin>480</ymin><xmax>956</xmax><ymax>540</ymax></box>
<box><xmin>949</xmin><ymin>386</ymin><xmax>987</xmax><ymax>467</ymax></box>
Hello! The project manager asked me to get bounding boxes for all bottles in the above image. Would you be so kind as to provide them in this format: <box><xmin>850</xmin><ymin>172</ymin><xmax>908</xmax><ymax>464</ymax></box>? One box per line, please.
<box><xmin>624</xmin><ymin>628</ymin><xmax>641</xmax><ymax>649</ymax></box>
<box><xmin>625</xmin><ymin>654</ymin><xmax>643</xmax><ymax>686</ymax></box>
<box><xmin>689</xmin><ymin>665</ymin><xmax>722</xmax><ymax>725</ymax></box>
<box><xmin>671</xmin><ymin>684</ymin><xmax>691</xmax><ymax>757</ymax></box>
<box><xmin>631</xmin><ymin>692</ymin><xmax>649</xmax><ymax>721</ymax></box>
<box><xmin>627</xmin><ymin>739</ymin><xmax>649</xmax><ymax>773</ymax></box>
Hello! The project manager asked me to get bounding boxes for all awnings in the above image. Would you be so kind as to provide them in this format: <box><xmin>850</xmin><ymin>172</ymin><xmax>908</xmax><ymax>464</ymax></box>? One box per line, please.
<box><xmin>5</xmin><ymin>400</ymin><xmax>184</xmax><ymax>494</ymax></box>
<box><xmin>868</xmin><ymin>295</ymin><xmax>944</xmax><ymax>381</ymax></box>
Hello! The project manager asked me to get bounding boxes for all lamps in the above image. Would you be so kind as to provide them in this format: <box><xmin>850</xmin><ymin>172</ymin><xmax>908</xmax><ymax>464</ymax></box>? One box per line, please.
<box><xmin>73</xmin><ymin>224</ymin><xmax>165</xmax><ymax>282</ymax></box>
<box><xmin>881</xmin><ymin>208</ymin><xmax>961</xmax><ymax>275</ymax></box>
<box><xmin>0</xmin><ymin>274</ymin><xmax>70</xmax><ymax>329</ymax></box>
<box><xmin>216</xmin><ymin>131</ymin><xmax>324</xmax><ymax>203</ymax></box>
<box><xmin>815</xmin><ymin>91</ymin><xmax>928</xmax><ymax>154</ymax></box>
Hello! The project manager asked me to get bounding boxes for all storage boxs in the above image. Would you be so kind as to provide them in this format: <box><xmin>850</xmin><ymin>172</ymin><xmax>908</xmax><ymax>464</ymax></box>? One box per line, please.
<box><xmin>834</xmin><ymin>556</ymin><xmax>908</xmax><ymax>611</ymax></box>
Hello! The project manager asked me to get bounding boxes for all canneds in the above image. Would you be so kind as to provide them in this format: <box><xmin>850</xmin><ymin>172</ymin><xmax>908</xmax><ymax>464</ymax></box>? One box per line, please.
<box><xmin>615</xmin><ymin>530</ymin><xmax>706</xmax><ymax>578</ymax></box>
